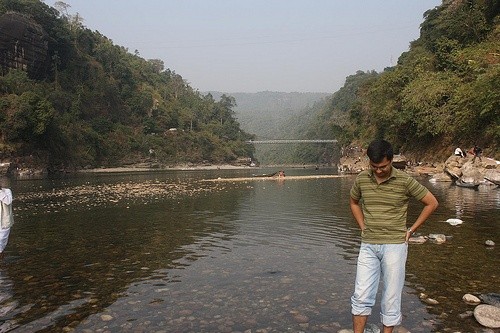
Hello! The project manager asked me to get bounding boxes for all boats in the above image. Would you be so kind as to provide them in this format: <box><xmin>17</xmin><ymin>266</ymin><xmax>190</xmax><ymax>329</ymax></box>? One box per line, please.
<box><xmin>455</xmin><ymin>178</ymin><xmax>481</xmax><ymax>188</ymax></box>
<box><xmin>484</xmin><ymin>176</ymin><xmax>500</xmax><ymax>188</ymax></box>
<box><xmin>251</xmin><ymin>171</ymin><xmax>278</xmax><ymax>177</ymax></box>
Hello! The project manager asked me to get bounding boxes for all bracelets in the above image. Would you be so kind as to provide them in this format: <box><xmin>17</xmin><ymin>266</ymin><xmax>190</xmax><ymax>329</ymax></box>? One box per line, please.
<box><xmin>408</xmin><ymin>228</ymin><xmax>413</xmax><ymax>234</ymax></box>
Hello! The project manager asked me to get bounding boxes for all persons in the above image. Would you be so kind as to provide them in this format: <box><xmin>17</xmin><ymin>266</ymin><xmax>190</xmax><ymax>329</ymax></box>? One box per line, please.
<box><xmin>455</xmin><ymin>145</ymin><xmax>467</xmax><ymax>159</ymax></box>
<box><xmin>0</xmin><ymin>174</ymin><xmax>14</xmax><ymax>255</ymax></box>
<box><xmin>350</xmin><ymin>138</ymin><xmax>439</xmax><ymax>333</ymax></box>
<box><xmin>471</xmin><ymin>145</ymin><xmax>483</xmax><ymax>164</ymax></box>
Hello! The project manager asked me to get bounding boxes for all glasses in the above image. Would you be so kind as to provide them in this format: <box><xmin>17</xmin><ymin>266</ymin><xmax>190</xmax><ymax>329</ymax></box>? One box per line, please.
<box><xmin>369</xmin><ymin>162</ymin><xmax>391</xmax><ymax>170</ymax></box>
